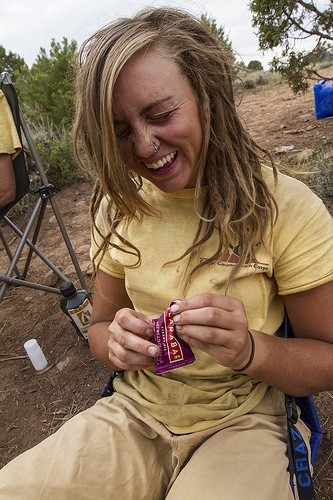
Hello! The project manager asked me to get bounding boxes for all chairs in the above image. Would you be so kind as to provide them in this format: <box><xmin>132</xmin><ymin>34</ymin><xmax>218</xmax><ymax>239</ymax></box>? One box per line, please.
<box><xmin>100</xmin><ymin>301</ymin><xmax>321</xmax><ymax>499</ymax></box>
<box><xmin>0</xmin><ymin>71</ymin><xmax>89</xmax><ymax>302</ymax></box>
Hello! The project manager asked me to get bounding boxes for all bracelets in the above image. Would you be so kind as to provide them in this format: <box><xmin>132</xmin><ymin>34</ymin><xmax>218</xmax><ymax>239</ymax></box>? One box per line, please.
<box><xmin>231</xmin><ymin>330</ymin><xmax>255</xmax><ymax>372</ymax></box>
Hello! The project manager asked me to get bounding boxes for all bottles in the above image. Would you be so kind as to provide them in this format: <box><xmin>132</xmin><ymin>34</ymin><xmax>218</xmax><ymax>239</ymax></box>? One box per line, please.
<box><xmin>59</xmin><ymin>281</ymin><xmax>93</xmax><ymax>347</ymax></box>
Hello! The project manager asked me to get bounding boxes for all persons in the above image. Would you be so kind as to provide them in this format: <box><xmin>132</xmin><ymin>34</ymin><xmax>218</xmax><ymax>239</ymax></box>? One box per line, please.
<box><xmin>0</xmin><ymin>4</ymin><xmax>333</xmax><ymax>500</ymax></box>
<box><xmin>0</xmin><ymin>89</ymin><xmax>22</xmax><ymax>210</ymax></box>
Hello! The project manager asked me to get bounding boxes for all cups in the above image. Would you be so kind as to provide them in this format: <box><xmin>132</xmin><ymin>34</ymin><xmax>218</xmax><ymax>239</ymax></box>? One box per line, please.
<box><xmin>24</xmin><ymin>339</ymin><xmax>47</xmax><ymax>370</ymax></box>
